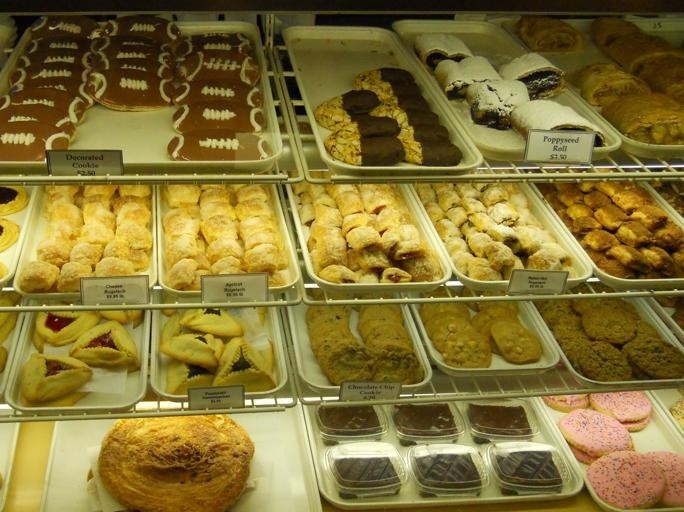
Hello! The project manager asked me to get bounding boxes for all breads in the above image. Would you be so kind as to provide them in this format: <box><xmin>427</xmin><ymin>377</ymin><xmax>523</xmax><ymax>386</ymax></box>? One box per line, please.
<box><xmin>0</xmin><ymin>87</ymin><xmax>86</xmax><ymax>126</ymax></box>
<box><xmin>115</xmin><ymin>221</ymin><xmax>152</xmax><ymax>249</ymax></box>
<box><xmin>177</xmin><ymin>32</ymin><xmax>255</xmax><ymax>55</ymax></box>
<box><xmin>203</xmin><ymin>238</ymin><xmax>243</xmax><ymax>262</ymax></box>
<box><xmin>591</xmin><ymin>392</ymin><xmax>652</xmax><ymax>424</ymax></box>
<box><xmin>22</xmin><ymin>354</ymin><xmax>92</xmax><ymax>403</ymax></box>
<box><xmin>99</xmin><ymin>309</ymin><xmax>145</xmax><ymax>329</ymax></box>
<box><xmin>8</xmin><ymin>61</ymin><xmax>92</xmax><ymax>86</ymax></box>
<box><xmin>0</xmin><ymin>103</ymin><xmax>76</xmax><ymax>145</ymax></box>
<box><xmin>234</xmin><ymin>185</ymin><xmax>267</xmax><ymax>200</ymax></box>
<box><xmin>100</xmin><ymin>415</ymin><xmax>255</xmax><ymax>512</ymax></box>
<box><xmin>96</xmin><ymin>257</ymin><xmax>136</xmax><ymax>276</ymax></box>
<box><xmin>96</xmin><ymin>36</ymin><xmax>175</xmax><ymax>54</ymax></box>
<box><xmin>51</xmin><ymin>204</ymin><xmax>84</xmax><ymax>226</ymax></box>
<box><xmin>235</xmin><ymin>198</ymin><xmax>271</xmax><ymax>220</ymax></box>
<box><xmin>244</xmin><ymin>230</ymin><xmax>280</xmax><ymax>249</ymax></box>
<box><xmin>79</xmin><ymin>223</ymin><xmax>114</xmax><ymax>245</ymax></box>
<box><xmin>369</xmin><ymin>92</ymin><xmax>439</xmax><ymax>127</ymax></box>
<box><xmin>560</xmin><ymin>410</ymin><xmax>635</xmax><ymax>460</ymax></box>
<box><xmin>545</xmin><ymin>394</ymin><xmax>589</xmax><ymax>412</ymax></box>
<box><xmin>184</xmin><ymin>270</ymin><xmax>211</xmax><ymax>291</ymax></box>
<box><xmin>114</xmin><ymin>196</ymin><xmax>151</xmax><ymax>223</ymax></box>
<box><xmin>166</xmin><ymin>236</ymin><xmax>205</xmax><ymax>265</ymax></box>
<box><xmin>68</xmin><ymin>321</ymin><xmax>139</xmax><ymax>373</ymax></box>
<box><xmin>180</xmin><ymin>307</ymin><xmax>245</xmax><ymax>338</ymax></box>
<box><xmin>37</xmin><ymin>302</ymin><xmax>99</xmax><ymax>345</ymax></box>
<box><xmin>172</xmin><ymin>99</ymin><xmax>267</xmax><ymax>134</ymax></box>
<box><xmin>602</xmin><ymin>33</ymin><xmax>682</xmax><ymax>73</ymax></box>
<box><xmin>45</xmin><ymin>223</ymin><xmax>82</xmax><ymax>241</ymax></box>
<box><xmin>591</xmin><ymin>17</ymin><xmax>644</xmax><ymax>50</ymax></box>
<box><xmin>44</xmin><ymin>193</ymin><xmax>73</xmax><ymax>217</ymax></box>
<box><xmin>71</xmin><ymin>243</ymin><xmax>104</xmax><ymax>268</ymax></box>
<box><xmin>103</xmin><ymin>14</ymin><xmax>181</xmax><ymax>40</ymax></box>
<box><xmin>585</xmin><ymin>451</ymin><xmax>665</xmax><ymax>510</ymax></box>
<box><xmin>163</xmin><ymin>258</ymin><xmax>197</xmax><ymax>289</ymax></box>
<box><xmin>168</xmin><ymin>128</ymin><xmax>274</xmax><ymax>161</ymax></box>
<box><xmin>199</xmin><ymin>201</ymin><xmax>235</xmax><ymax>221</ymax></box>
<box><xmin>212</xmin><ymin>335</ymin><xmax>277</xmax><ymax>391</ymax></box>
<box><xmin>324</xmin><ymin>115</ymin><xmax>406</xmax><ymax>166</ymax></box>
<box><xmin>89</xmin><ymin>58</ymin><xmax>177</xmax><ymax>80</ymax></box>
<box><xmin>92</xmin><ymin>45</ymin><xmax>173</xmax><ymax>67</ymax></box>
<box><xmin>513</xmin><ymin>16</ymin><xmax>583</xmax><ymax>54</ymax></box>
<box><xmin>415</xmin><ymin>179</ymin><xmax>577</xmax><ymax>281</ymax></box>
<box><xmin>86</xmin><ymin>68</ymin><xmax>175</xmax><ymax>111</ymax></box>
<box><xmin>103</xmin><ymin>239</ymin><xmax>150</xmax><ymax>272</ymax></box>
<box><xmin>16</xmin><ymin>49</ymin><xmax>93</xmax><ymax>69</ymax></box>
<box><xmin>314</xmin><ymin>88</ymin><xmax>382</xmax><ymax>132</ymax></box>
<box><xmin>159</xmin><ymin>332</ymin><xmax>223</xmax><ymax>368</ymax></box>
<box><xmin>622</xmin><ymin>416</ymin><xmax>651</xmax><ymax>433</ymax></box>
<box><xmin>210</xmin><ymin>258</ymin><xmax>240</xmax><ymax>275</ymax></box>
<box><xmin>572</xmin><ymin>62</ymin><xmax>651</xmax><ymax>107</ymax></box>
<box><xmin>0</xmin><ymin>120</ymin><xmax>70</xmax><ymax>161</ymax></box>
<box><xmin>57</xmin><ymin>263</ymin><xmax>92</xmax><ymax>293</ymax></box>
<box><xmin>167</xmin><ymin>357</ymin><xmax>215</xmax><ymax>393</ymax></box>
<box><xmin>641</xmin><ymin>56</ymin><xmax>684</xmax><ymax>92</ymax></box>
<box><xmin>528</xmin><ymin>167</ymin><xmax>684</xmax><ymax>278</ymax></box>
<box><xmin>84</xmin><ymin>202</ymin><xmax>116</xmax><ymax>229</ymax></box>
<box><xmin>201</xmin><ymin>189</ymin><xmax>231</xmax><ymax>204</ymax></box>
<box><xmin>9</xmin><ymin>75</ymin><xmax>97</xmax><ymax>109</ymax></box>
<box><xmin>202</xmin><ymin>215</ymin><xmax>239</xmax><ymax>242</ymax></box>
<box><xmin>83</xmin><ymin>185</ymin><xmax>119</xmax><ymax>196</ymax></box>
<box><xmin>243</xmin><ymin>244</ymin><xmax>279</xmax><ymax>275</ymax></box>
<box><xmin>172</xmin><ymin>77</ymin><xmax>263</xmax><ymax>110</ymax></box>
<box><xmin>291</xmin><ymin>169</ymin><xmax>444</xmax><ymax>283</ymax></box>
<box><xmin>240</xmin><ymin>215</ymin><xmax>277</xmax><ymax>237</ymax></box>
<box><xmin>163</xmin><ymin>208</ymin><xmax>201</xmax><ymax>234</ymax></box>
<box><xmin>603</xmin><ymin>91</ymin><xmax>683</xmax><ymax>146</ymax></box>
<box><xmin>29</xmin><ymin>16</ymin><xmax>105</xmax><ymax>44</ymax></box>
<box><xmin>37</xmin><ymin>237</ymin><xmax>71</xmax><ymax>266</ymax></box>
<box><xmin>24</xmin><ymin>35</ymin><xmax>92</xmax><ymax>53</ymax></box>
<box><xmin>569</xmin><ymin>442</ymin><xmax>596</xmax><ymax>465</ymax></box>
<box><xmin>47</xmin><ymin>185</ymin><xmax>80</xmax><ymax>196</ymax></box>
<box><xmin>0</xmin><ymin>217</ymin><xmax>20</xmax><ymax>252</ymax></box>
<box><xmin>163</xmin><ymin>184</ymin><xmax>200</xmax><ymax>206</ymax></box>
<box><xmin>396</xmin><ymin>124</ymin><xmax>463</xmax><ymax>166</ymax></box>
<box><xmin>191</xmin><ymin>255</ymin><xmax>212</xmax><ymax>271</ymax></box>
<box><xmin>353</xmin><ymin>67</ymin><xmax>421</xmax><ymax>102</ymax></box>
<box><xmin>173</xmin><ymin>48</ymin><xmax>261</xmax><ymax>86</ymax></box>
<box><xmin>0</xmin><ymin>184</ymin><xmax>30</xmax><ymax>215</ymax></box>
<box><xmin>77</xmin><ymin>194</ymin><xmax>111</xmax><ymax>210</ymax></box>
<box><xmin>19</xmin><ymin>261</ymin><xmax>60</xmax><ymax>293</ymax></box>
<box><xmin>648</xmin><ymin>450</ymin><xmax>684</xmax><ymax>508</ymax></box>
<box><xmin>118</xmin><ymin>185</ymin><xmax>152</xmax><ymax>199</ymax></box>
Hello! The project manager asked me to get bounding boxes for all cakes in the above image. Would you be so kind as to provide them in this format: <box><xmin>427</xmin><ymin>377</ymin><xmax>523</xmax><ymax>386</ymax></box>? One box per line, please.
<box><xmin>510</xmin><ymin>99</ymin><xmax>606</xmax><ymax>147</ymax></box>
<box><xmin>497</xmin><ymin>52</ymin><xmax>565</xmax><ymax>102</ymax></box>
<box><xmin>434</xmin><ymin>55</ymin><xmax>503</xmax><ymax>103</ymax></box>
<box><xmin>414</xmin><ymin>34</ymin><xmax>474</xmax><ymax>75</ymax></box>
<box><xmin>466</xmin><ymin>79</ymin><xmax>530</xmax><ymax>130</ymax></box>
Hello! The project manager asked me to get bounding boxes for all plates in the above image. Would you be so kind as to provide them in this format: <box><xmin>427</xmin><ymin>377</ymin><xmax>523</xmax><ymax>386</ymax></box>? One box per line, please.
<box><xmin>4</xmin><ymin>310</ymin><xmax>151</xmax><ymax>415</ymax></box>
<box><xmin>501</xmin><ymin>17</ymin><xmax>683</xmax><ymax>160</ymax></box>
<box><xmin>299</xmin><ymin>399</ymin><xmax>585</xmax><ymax>512</ymax></box>
<box><xmin>285</xmin><ymin>289</ymin><xmax>433</xmax><ymax>395</ymax></box>
<box><xmin>1</xmin><ymin>185</ymin><xmax>40</xmax><ymax>286</ymax></box>
<box><xmin>529</xmin><ymin>181</ymin><xmax>684</xmax><ymax>289</ymax></box>
<box><xmin>404</xmin><ymin>290</ymin><xmax>561</xmax><ymax>380</ymax></box>
<box><xmin>282</xmin><ymin>25</ymin><xmax>485</xmax><ymax>175</ymax></box>
<box><xmin>407</xmin><ymin>183</ymin><xmax>594</xmax><ymax>291</ymax></box>
<box><xmin>13</xmin><ymin>182</ymin><xmax>159</xmax><ymax>300</ymax></box>
<box><xmin>285</xmin><ymin>184</ymin><xmax>453</xmax><ymax>294</ymax></box>
<box><xmin>39</xmin><ymin>400</ymin><xmax>322</xmax><ymax>512</ymax></box>
<box><xmin>527</xmin><ymin>298</ymin><xmax>684</xmax><ymax>388</ymax></box>
<box><xmin>537</xmin><ymin>388</ymin><xmax>684</xmax><ymax>512</ymax></box>
<box><xmin>148</xmin><ymin>309</ymin><xmax>287</xmax><ymax>402</ymax></box>
<box><xmin>156</xmin><ymin>186</ymin><xmax>301</xmax><ymax>297</ymax></box>
<box><xmin>0</xmin><ymin>19</ymin><xmax>283</xmax><ymax>173</ymax></box>
<box><xmin>393</xmin><ymin>19</ymin><xmax>623</xmax><ymax>161</ymax></box>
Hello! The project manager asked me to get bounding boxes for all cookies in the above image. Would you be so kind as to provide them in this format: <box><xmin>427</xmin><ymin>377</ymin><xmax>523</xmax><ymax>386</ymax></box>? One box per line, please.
<box><xmin>532</xmin><ymin>282</ymin><xmax>684</xmax><ymax>381</ymax></box>
<box><xmin>304</xmin><ymin>286</ymin><xmax>423</xmax><ymax>384</ymax></box>
<box><xmin>418</xmin><ymin>287</ymin><xmax>540</xmax><ymax>369</ymax></box>
